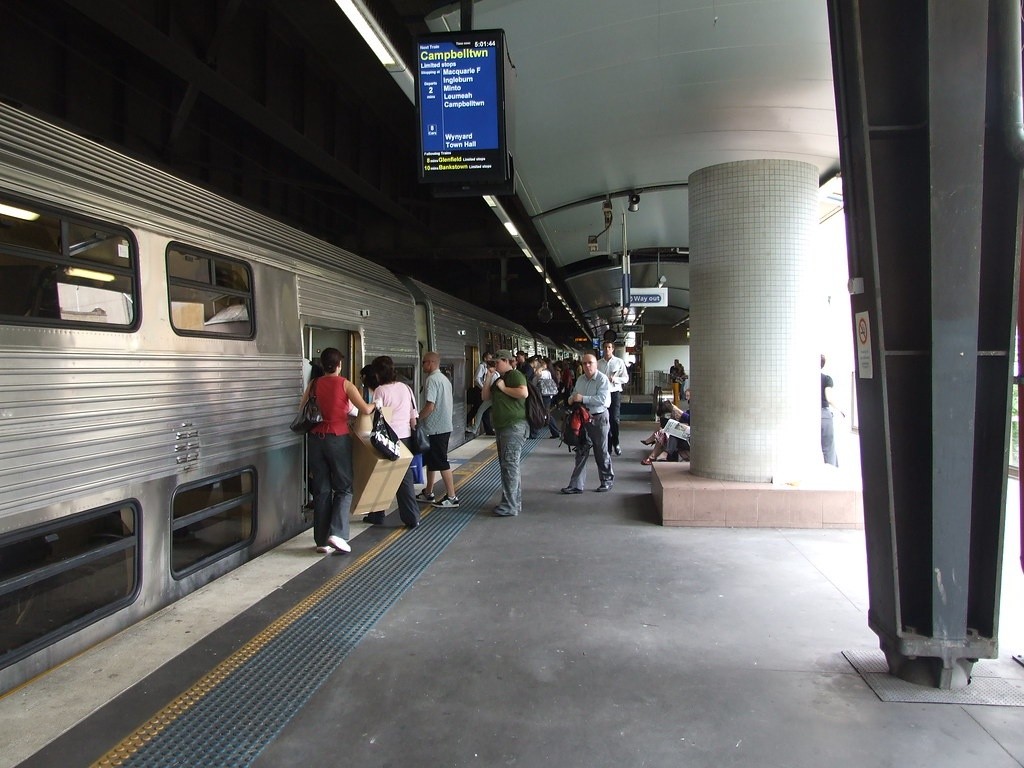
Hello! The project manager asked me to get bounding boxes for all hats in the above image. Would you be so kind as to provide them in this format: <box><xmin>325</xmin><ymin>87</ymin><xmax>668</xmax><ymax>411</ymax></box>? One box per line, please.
<box><xmin>490</xmin><ymin>349</ymin><xmax>512</xmax><ymax>362</ymax></box>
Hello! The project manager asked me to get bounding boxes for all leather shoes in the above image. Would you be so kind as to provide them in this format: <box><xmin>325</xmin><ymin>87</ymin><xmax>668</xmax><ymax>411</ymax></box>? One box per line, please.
<box><xmin>560</xmin><ymin>486</ymin><xmax>583</xmax><ymax>493</ymax></box>
<box><xmin>614</xmin><ymin>445</ymin><xmax>621</xmax><ymax>455</ymax></box>
<box><xmin>596</xmin><ymin>484</ymin><xmax>614</xmax><ymax>491</ymax></box>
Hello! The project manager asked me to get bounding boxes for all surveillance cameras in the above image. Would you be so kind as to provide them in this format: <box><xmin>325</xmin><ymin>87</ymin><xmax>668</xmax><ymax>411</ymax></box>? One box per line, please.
<box><xmin>588</xmin><ymin>235</ymin><xmax>598</xmax><ymax>253</ymax></box>
<box><xmin>537</xmin><ymin>307</ymin><xmax>553</xmax><ymax>323</ymax></box>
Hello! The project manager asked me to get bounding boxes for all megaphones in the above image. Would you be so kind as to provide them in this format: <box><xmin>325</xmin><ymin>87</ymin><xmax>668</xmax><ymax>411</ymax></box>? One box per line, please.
<box><xmin>628</xmin><ymin>194</ymin><xmax>640</xmax><ymax>212</ymax></box>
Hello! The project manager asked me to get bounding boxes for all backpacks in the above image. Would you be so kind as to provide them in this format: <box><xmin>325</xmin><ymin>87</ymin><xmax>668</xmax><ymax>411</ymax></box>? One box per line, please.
<box><xmin>488</xmin><ymin>371</ymin><xmax>547</xmax><ymax>429</ymax></box>
<box><xmin>560</xmin><ymin>403</ymin><xmax>594</xmax><ymax>454</ymax></box>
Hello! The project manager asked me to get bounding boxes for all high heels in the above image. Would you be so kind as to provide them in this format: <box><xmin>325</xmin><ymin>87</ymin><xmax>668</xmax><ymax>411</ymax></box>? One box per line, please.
<box><xmin>641</xmin><ymin>440</ymin><xmax>656</xmax><ymax>446</ymax></box>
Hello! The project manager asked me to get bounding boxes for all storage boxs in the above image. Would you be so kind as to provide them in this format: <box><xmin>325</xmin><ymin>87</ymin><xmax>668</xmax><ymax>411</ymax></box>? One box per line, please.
<box><xmin>348</xmin><ymin>406</ymin><xmax>414</xmax><ymax>516</ymax></box>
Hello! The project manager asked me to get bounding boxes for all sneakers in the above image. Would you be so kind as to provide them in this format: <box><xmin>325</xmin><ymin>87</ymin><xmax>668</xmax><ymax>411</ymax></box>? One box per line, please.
<box><xmin>431</xmin><ymin>493</ymin><xmax>459</xmax><ymax>507</ymax></box>
<box><xmin>416</xmin><ymin>488</ymin><xmax>435</xmax><ymax>502</ymax></box>
<box><xmin>316</xmin><ymin>545</ymin><xmax>336</xmax><ymax>553</ymax></box>
<box><xmin>328</xmin><ymin>535</ymin><xmax>351</xmax><ymax>552</ymax></box>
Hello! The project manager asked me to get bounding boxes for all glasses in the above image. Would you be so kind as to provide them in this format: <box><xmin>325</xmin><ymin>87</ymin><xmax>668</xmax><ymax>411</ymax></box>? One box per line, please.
<box><xmin>423</xmin><ymin>360</ymin><xmax>434</xmax><ymax>364</ymax></box>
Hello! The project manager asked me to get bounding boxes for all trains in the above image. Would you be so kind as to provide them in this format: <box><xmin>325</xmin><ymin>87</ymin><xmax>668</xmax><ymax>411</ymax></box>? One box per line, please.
<box><xmin>0</xmin><ymin>103</ymin><xmax>585</xmax><ymax>698</ymax></box>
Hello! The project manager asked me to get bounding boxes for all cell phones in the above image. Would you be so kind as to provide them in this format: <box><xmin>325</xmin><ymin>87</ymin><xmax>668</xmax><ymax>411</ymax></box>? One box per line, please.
<box><xmin>486</xmin><ymin>360</ymin><xmax>497</xmax><ymax>367</ymax></box>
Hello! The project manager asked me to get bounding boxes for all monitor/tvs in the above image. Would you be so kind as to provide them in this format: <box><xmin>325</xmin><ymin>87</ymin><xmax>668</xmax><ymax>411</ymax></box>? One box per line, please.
<box><xmin>415</xmin><ymin>28</ymin><xmax>509</xmax><ymax>183</ymax></box>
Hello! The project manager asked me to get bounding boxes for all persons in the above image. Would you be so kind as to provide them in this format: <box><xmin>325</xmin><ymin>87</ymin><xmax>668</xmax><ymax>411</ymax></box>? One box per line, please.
<box><xmin>821</xmin><ymin>353</ymin><xmax>845</xmax><ymax>468</ymax></box>
<box><xmin>670</xmin><ymin>359</ymin><xmax>687</xmax><ymax>400</ymax></box>
<box><xmin>298</xmin><ymin>347</ymin><xmax>459</xmax><ymax>553</ymax></box>
<box><xmin>466</xmin><ymin>340</ymin><xmax>629</xmax><ymax>516</ymax></box>
<box><xmin>641</xmin><ymin>388</ymin><xmax>690</xmax><ymax>465</ymax></box>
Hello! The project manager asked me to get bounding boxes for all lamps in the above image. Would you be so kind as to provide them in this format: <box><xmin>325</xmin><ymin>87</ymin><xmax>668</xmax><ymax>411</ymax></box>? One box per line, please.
<box><xmin>632</xmin><ymin>276</ymin><xmax>667</xmax><ymax>326</ymax></box>
<box><xmin>482</xmin><ymin>194</ymin><xmax>593</xmax><ymax>344</ymax></box>
<box><xmin>62</xmin><ymin>266</ymin><xmax>118</xmax><ymax>282</ymax></box>
<box><xmin>336</xmin><ymin>0</ymin><xmax>414</xmax><ymax>105</ymax></box>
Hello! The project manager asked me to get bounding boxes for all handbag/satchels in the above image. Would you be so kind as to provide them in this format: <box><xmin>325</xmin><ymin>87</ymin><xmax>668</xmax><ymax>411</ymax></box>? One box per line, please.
<box><xmin>468</xmin><ymin>387</ymin><xmax>483</xmax><ymax>404</ymax></box>
<box><xmin>289</xmin><ymin>398</ymin><xmax>323</xmax><ymax>435</ymax></box>
<box><xmin>370</xmin><ymin>407</ymin><xmax>401</xmax><ymax>461</ymax></box>
<box><xmin>409</xmin><ymin>418</ymin><xmax>431</xmax><ymax>454</ymax></box>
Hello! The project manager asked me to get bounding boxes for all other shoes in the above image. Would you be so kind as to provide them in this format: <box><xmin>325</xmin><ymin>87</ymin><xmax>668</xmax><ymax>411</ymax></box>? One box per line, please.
<box><xmin>641</xmin><ymin>458</ymin><xmax>657</xmax><ymax>465</ymax></box>
<box><xmin>493</xmin><ymin>507</ymin><xmax>512</xmax><ymax>516</ymax></box>
<box><xmin>486</xmin><ymin>431</ymin><xmax>495</xmax><ymax>435</ymax></box>
<box><xmin>465</xmin><ymin>426</ymin><xmax>476</xmax><ymax>434</ymax></box>
<box><xmin>406</xmin><ymin>519</ymin><xmax>419</xmax><ymax>526</ymax></box>
<box><xmin>363</xmin><ymin>516</ymin><xmax>383</xmax><ymax>524</ymax></box>
